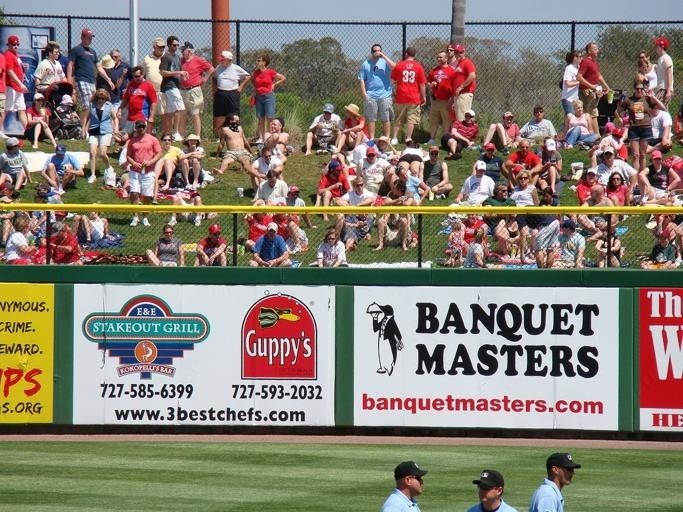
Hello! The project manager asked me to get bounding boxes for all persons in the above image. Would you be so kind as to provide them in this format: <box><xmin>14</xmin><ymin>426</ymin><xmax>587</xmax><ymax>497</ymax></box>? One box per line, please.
<box><xmin>465</xmin><ymin>469</ymin><xmax>518</xmax><ymax>512</ymax></box>
<box><xmin>528</xmin><ymin>453</ymin><xmax>581</xmax><ymax>512</ymax></box>
<box><xmin>380</xmin><ymin>461</ymin><xmax>428</xmax><ymax>512</ymax></box>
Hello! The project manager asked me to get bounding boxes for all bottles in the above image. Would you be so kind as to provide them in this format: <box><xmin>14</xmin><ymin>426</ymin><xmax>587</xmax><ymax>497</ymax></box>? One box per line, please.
<box><xmin>238</xmin><ymin>242</ymin><xmax>244</xmax><ymax>257</ymax></box>
<box><xmin>509</xmin><ymin>246</ymin><xmax>515</xmax><ymax>258</ymax></box>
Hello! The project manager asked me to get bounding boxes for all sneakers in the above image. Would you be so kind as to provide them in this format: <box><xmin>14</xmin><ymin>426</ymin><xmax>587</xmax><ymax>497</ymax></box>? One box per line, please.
<box><xmin>142</xmin><ymin>217</ymin><xmax>150</xmax><ymax>226</ymax></box>
<box><xmin>168</xmin><ymin>220</ymin><xmax>177</xmax><ymax>227</ymax></box>
<box><xmin>195</xmin><ymin>218</ymin><xmax>201</xmax><ymax>226</ymax></box>
<box><xmin>130</xmin><ymin>216</ymin><xmax>139</xmax><ymax>225</ymax></box>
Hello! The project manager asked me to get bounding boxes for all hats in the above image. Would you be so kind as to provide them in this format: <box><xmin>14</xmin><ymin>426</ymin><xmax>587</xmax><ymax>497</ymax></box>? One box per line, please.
<box><xmin>267</xmin><ymin>222</ymin><xmax>279</xmax><ymax>232</ymax></box>
<box><xmin>447</xmin><ymin>44</ymin><xmax>464</xmax><ymax>53</ymax></box>
<box><xmin>394</xmin><ymin>460</ymin><xmax>427</xmax><ymax>479</ymax></box>
<box><xmin>560</xmin><ymin>219</ymin><xmax>576</xmax><ymax>230</ymax></box>
<box><xmin>288</xmin><ymin>185</ymin><xmax>300</xmax><ymax>193</ymax></box>
<box><xmin>322</xmin><ymin>103</ymin><xmax>441</xmax><ymax>173</ymax></box>
<box><xmin>209</xmin><ymin>224</ymin><xmax>222</xmax><ymax>233</ymax></box>
<box><xmin>654</xmin><ymin>36</ymin><xmax>670</xmax><ymax>49</ymax></box>
<box><xmin>6</xmin><ymin>28</ymin><xmax>234</xmax><ymax>155</ymax></box>
<box><xmin>464</xmin><ymin>111</ymin><xmax>662</xmax><ymax>171</ymax></box>
<box><xmin>472</xmin><ymin>470</ymin><xmax>504</xmax><ymax>488</ymax></box>
<box><xmin>547</xmin><ymin>453</ymin><xmax>581</xmax><ymax>470</ymax></box>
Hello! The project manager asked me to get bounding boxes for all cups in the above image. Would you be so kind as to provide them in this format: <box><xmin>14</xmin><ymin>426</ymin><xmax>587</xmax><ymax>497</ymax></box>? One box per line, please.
<box><xmin>236</xmin><ymin>188</ymin><xmax>243</xmax><ymax>197</ymax></box>
<box><xmin>428</xmin><ymin>189</ymin><xmax>434</xmax><ymax>200</ymax></box>
<box><xmin>607</xmin><ymin>91</ymin><xmax>613</xmax><ymax>104</ymax></box>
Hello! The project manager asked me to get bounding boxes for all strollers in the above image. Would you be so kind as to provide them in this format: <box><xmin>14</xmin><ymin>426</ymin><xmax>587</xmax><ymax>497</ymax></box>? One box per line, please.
<box><xmin>42</xmin><ymin>82</ymin><xmax>81</xmax><ymax>140</ymax></box>
